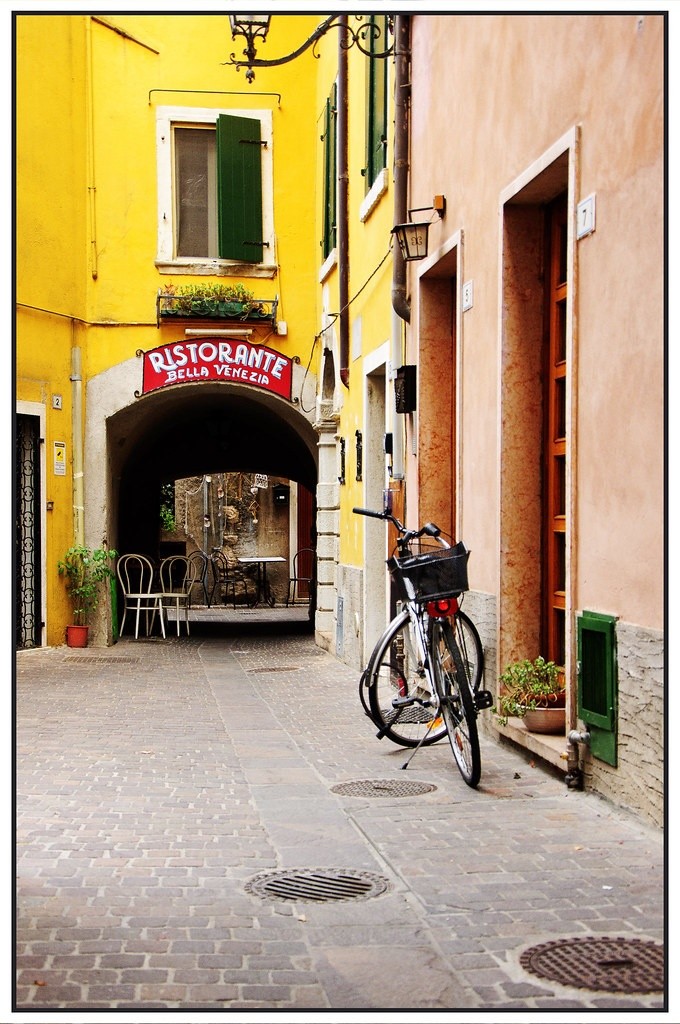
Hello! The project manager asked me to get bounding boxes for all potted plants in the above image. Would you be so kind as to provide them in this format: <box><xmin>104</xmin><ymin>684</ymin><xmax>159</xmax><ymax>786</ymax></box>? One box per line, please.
<box><xmin>488</xmin><ymin>655</ymin><xmax>565</xmax><ymax>736</ymax></box>
<box><xmin>57</xmin><ymin>543</ymin><xmax>119</xmax><ymax>648</ymax></box>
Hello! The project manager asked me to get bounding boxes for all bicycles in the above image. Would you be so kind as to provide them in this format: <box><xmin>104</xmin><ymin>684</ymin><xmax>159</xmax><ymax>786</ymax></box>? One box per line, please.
<box><xmin>351</xmin><ymin>508</ymin><xmax>494</xmax><ymax>788</ymax></box>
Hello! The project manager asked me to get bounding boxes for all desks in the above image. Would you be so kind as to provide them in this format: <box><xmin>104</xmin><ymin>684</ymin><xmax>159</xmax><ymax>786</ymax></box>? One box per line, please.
<box><xmin>237</xmin><ymin>556</ymin><xmax>287</xmax><ymax>609</ymax></box>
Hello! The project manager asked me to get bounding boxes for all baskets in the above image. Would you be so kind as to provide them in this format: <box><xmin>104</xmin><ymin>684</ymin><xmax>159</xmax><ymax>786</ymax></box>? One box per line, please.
<box><xmin>387</xmin><ymin>541</ymin><xmax>470</xmax><ymax>603</ymax></box>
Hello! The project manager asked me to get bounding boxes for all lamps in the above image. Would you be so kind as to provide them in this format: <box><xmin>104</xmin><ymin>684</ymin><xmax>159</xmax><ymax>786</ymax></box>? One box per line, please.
<box><xmin>219</xmin><ymin>15</ymin><xmax>396</xmax><ymax>84</ymax></box>
<box><xmin>390</xmin><ymin>195</ymin><xmax>446</xmax><ymax>261</ymax></box>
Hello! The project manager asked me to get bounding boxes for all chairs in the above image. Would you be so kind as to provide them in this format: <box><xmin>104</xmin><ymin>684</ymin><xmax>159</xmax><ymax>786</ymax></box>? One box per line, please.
<box><xmin>116</xmin><ymin>554</ymin><xmax>166</xmax><ymax>640</ymax></box>
<box><xmin>149</xmin><ymin>556</ymin><xmax>196</xmax><ymax>636</ymax></box>
<box><xmin>169</xmin><ymin>550</ymin><xmax>210</xmax><ymax>610</ymax></box>
<box><xmin>285</xmin><ymin>548</ymin><xmax>314</xmax><ymax>608</ymax></box>
<box><xmin>209</xmin><ymin>551</ymin><xmax>249</xmax><ymax>611</ymax></box>
